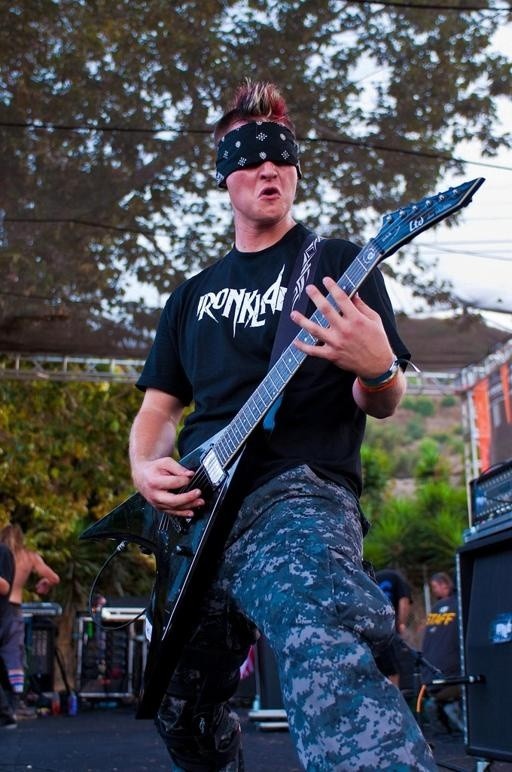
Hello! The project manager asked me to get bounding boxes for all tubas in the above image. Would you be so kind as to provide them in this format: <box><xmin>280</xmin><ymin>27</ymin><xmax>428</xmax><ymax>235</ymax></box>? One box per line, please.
<box><xmin>81</xmin><ymin>178</ymin><xmax>485</xmax><ymax>646</ymax></box>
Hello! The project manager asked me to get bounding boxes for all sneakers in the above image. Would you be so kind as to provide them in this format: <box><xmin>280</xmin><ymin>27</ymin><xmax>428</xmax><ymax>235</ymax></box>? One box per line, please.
<box><xmin>0</xmin><ymin>700</ymin><xmax>38</xmax><ymax>729</ymax></box>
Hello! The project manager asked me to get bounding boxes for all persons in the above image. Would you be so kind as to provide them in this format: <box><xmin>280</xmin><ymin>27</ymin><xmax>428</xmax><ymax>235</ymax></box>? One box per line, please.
<box><xmin>129</xmin><ymin>77</ymin><xmax>439</xmax><ymax>772</ymax></box>
<box><xmin>0</xmin><ymin>525</ymin><xmax>60</xmax><ymax>730</ymax></box>
<box><xmin>375</xmin><ymin>570</ymin><xmax>460</xmax><ymax>733</ymax></box>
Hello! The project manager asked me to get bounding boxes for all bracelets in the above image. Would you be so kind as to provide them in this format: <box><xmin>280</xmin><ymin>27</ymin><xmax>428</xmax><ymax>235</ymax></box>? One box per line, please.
<box><xmin>357</xmin><ymin>355</ymin><xmax>421</xmax><ymax>393</ymax></box>
